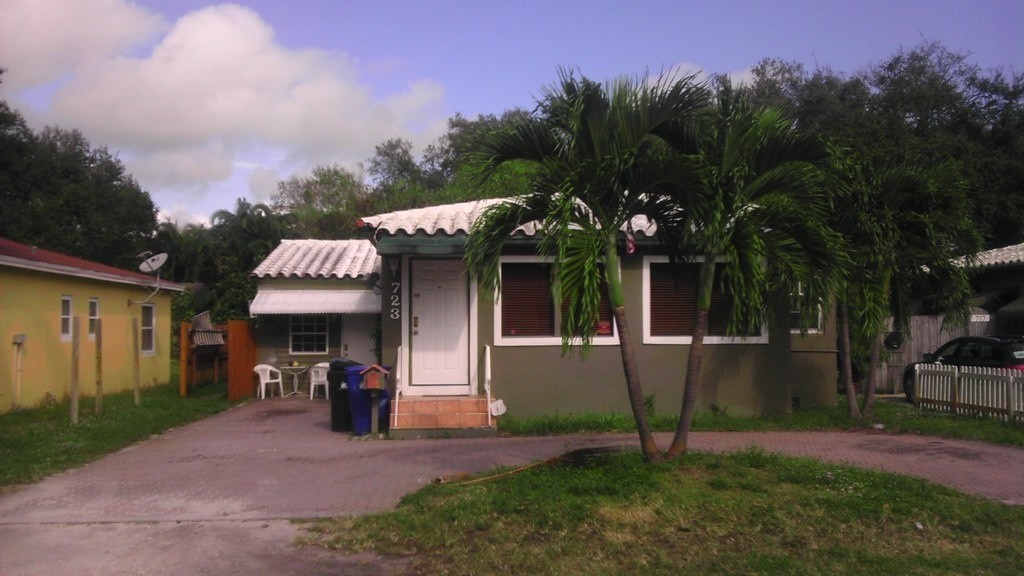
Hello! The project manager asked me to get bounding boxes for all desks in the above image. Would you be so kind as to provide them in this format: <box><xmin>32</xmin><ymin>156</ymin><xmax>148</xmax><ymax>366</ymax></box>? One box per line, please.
<box><xmin>279</xmin><ymin>366</ymin><xmax>310</xmax><ymax>398</ymax></box>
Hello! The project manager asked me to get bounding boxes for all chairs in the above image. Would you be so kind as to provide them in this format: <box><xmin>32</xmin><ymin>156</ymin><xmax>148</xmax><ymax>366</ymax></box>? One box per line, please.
<box><xmin>253</xmin><ymin>363</ymin><xmax>283</xmax><ymax>399</ymax></box>
<box><xmin>309</xmin><ymin>365</ymin><xmax>330</xmax><ymax>400</ymax></box>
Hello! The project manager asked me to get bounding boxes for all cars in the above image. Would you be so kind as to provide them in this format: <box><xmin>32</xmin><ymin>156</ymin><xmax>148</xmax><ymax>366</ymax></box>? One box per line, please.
<box><xmin>903</xmin><ymin>335</ymin><xmax>1024</xmax><ymax>403</ymax></box>
